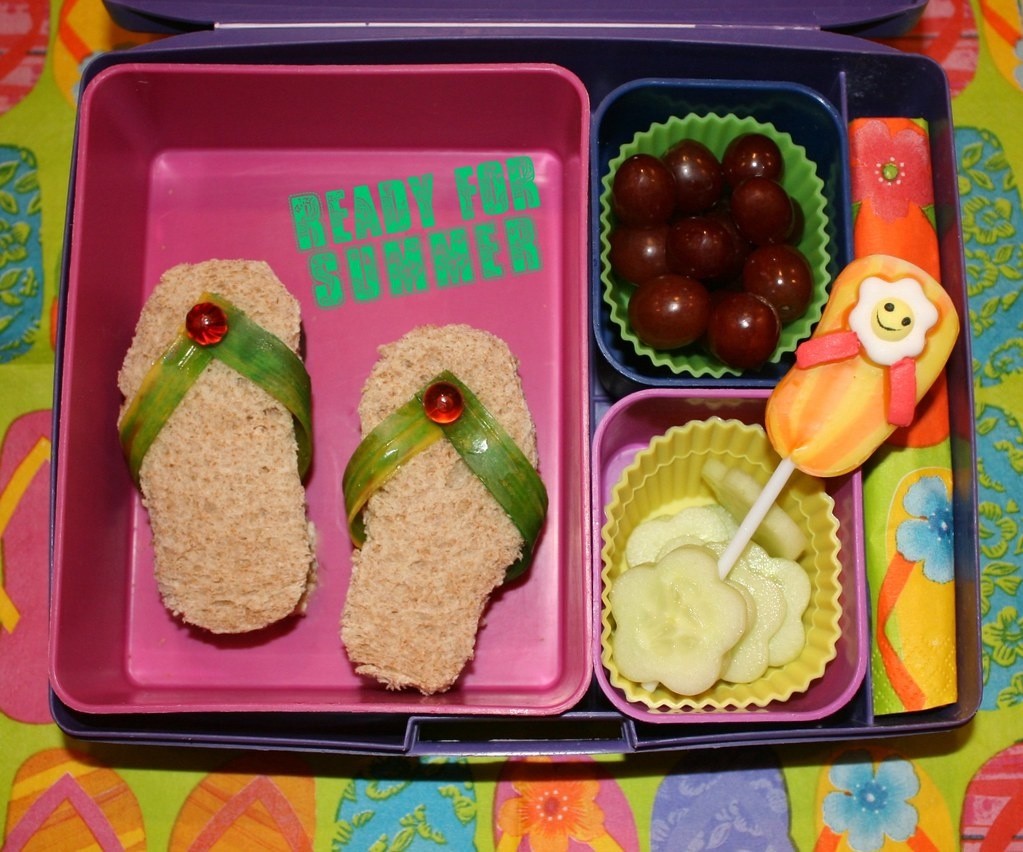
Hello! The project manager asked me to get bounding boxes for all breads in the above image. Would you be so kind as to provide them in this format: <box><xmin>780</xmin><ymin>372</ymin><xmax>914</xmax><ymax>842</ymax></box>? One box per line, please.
<box><xmin>119</xmin><ymin>258</ymin><xmax>540</xmax><ymax>694</ymax></box>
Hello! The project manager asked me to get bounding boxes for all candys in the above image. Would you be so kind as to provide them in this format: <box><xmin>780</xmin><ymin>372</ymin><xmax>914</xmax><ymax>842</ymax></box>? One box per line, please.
<box><xmin>764</xmin><ymin>254</ymin><xmax>961</xmax><ymax>477</ymax></box>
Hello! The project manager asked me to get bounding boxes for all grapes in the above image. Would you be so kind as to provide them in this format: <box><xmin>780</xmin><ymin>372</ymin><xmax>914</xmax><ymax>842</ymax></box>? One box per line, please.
<box><xmin>611</xmin><ymin>132</ymin><xmax>813</xmax><ymax>367</ymax></box>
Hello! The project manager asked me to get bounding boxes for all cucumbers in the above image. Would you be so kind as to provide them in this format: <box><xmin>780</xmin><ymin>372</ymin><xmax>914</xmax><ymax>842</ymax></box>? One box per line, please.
<box><xmin>610</xmin><ymin>457</ymin><xmax>812</xmax><ymax>696</ymax></box>
